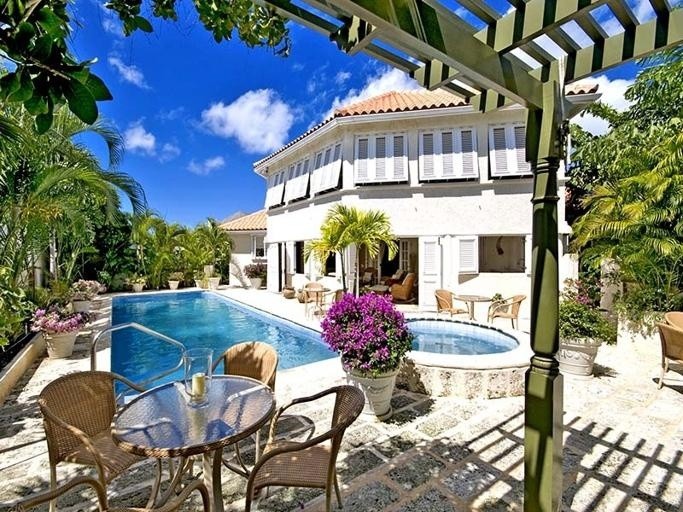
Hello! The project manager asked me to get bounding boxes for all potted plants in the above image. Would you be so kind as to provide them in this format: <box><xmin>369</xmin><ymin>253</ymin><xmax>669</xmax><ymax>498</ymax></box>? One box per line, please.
<box><xmin>208</xmin><ymin>271</ymin><xmax>222</xmax><ymax>290</ymax></box>
<box><xmin>164</xmin><ymin>271</ymin><xmax>183</xmax><ymax>290</ymax></box>
<box><xmin>192</xmin><ymin>271</ymin><xmax>205</xmax><ymax>287</ymax></box>
<box><xmin>96</xmin><ymin>269</ymin><xmax>113</xmax><ymax>294</ymax></box>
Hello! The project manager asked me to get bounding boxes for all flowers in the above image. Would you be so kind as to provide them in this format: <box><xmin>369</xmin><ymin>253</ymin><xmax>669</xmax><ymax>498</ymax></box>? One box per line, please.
<box><xmin>127</xmin><ymin>272</ymin><xmax>150</xmax><ymax>284</ymax></box>
<box><xmin>558</xmin><ymin>274</ymin><xmax>622</xmax><ymax>344</ymax></box>
<box><xmin>243</xmin><ymin>262</ymin><xmax>270</xmax><ymax>279</ymax></box>
<box><xmin>28</xmin><ymin>304</ymin><xmax>95</xmax><ymax>336</ymax></box>
<box><xmin>318</xmin><ymin>292</ymin><xmax>417</xmax><ymax>378</ymax></box>
<box><xmin>67</xmin><ymin>278</ymin><xmax>107</xmax><ymax>300</ymax></box>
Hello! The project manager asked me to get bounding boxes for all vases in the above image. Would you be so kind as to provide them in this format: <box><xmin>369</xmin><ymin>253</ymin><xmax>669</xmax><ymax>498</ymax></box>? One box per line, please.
<box><xmin>282</xmin><ymin>287</ymin><xmax>295</xmax><ymax>299</ymax></box>
<box><xmin>557</xmin><ymin>332</ymin><xmax>605</xmax><ymax>379</ymax></box>
<box><xmin>72</xmin><ymin>300</ymin><xmax>91</xmax><ymax>314</ymax></box>
<box><xmin>295</xmin><ymin>290</ymin><xmax>308</xmax><ymax>303</ymax></box>
<box><xmin>343</xmin><ymin>361</ymin><xmax>406</xmax><ymax>425</ymax></box>
<box><xmin>250</xmin><ymin>276</ymin><xmax>262</xmax><ymax>289</ymax></box>
<box><xmin>132</xmin><ymin>285</ymin><xmax>144</xmax><ymax>294</ymax></box>
<box><xmin>44</xmin><ymin>330</ymin><xmax>77</xmax><ymax>361</ymax></box>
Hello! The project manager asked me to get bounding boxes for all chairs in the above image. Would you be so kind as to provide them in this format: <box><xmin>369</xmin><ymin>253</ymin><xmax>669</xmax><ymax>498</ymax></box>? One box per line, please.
<box><xmin>302</xmin><ymin>280</ymin><xmax>326</xmax><ymax>315</ymax></box>
<box><xmin>195</xmin><ymin>340</ymin><xmax>285</xmax><ymax>485</ymax></box>
<box><xmin>652</xmin><ymin>319</ymin><xmax>682</xmax><ymax>391</ymax></box>
<box><xmin>241</xmin><ymin>379</ymin><xmax>365</xmax><ymax>512</ymax></box>
<box><xmin>7</xmin><ymin>475</ymin><xmax>214</xmax><ymax>512</ymax></box>
<box><xmin>359</xmin><ymin>266</ymin><xmax>418</xmax><ymax>304</ymax></box>
<box><xmin>486</xmin><ymin>294</ymin><xmax>528</xmax><ymax>330</ymax></box>
<box><xmin>34</xmin><ymin>373</ymin><xmax>186</xmax><ymax>512</ymax></box>
<box><xmin>431</xmin><ymin>287</ymin><xmax>467</xmax><ymax>320</ymax></box>
<box><xmin>317</xmin><ymin>285</ymin><xmax>347</xmax><ymax>318</ymax></box>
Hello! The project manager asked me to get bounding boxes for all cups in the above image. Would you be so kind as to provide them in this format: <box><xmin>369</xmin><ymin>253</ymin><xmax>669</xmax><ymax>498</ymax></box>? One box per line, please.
<box><xmin>182</xmin><ymin>347</ymin><xmax>213</xmax><ymax>408</ymax></box>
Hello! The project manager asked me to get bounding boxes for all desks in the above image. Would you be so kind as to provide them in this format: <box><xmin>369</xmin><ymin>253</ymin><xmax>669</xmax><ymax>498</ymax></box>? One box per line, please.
<box><xmin>104</xmin><ymin>372</ymin><xmax>278</xmax><ymax>511</ymax></box>
<box><xmin>450</xmin><ymin>295</ymin><xmax>491</xmax><ymax>321</ymax></box>
<box><xmin>304</xmin><ymin>287</ymin><xmax>328</xmax><ymax>316</ymax></box>
<box><xmin>664</xmin><ymin>311</ymin><xmax>683</xmax><ymax>334</ymax></box>
